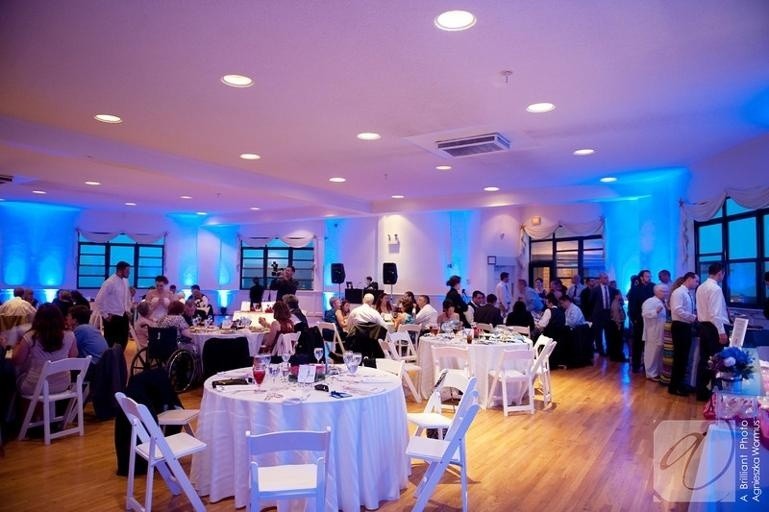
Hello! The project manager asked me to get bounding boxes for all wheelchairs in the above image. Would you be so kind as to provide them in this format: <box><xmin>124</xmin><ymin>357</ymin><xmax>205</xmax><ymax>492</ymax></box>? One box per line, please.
<box><xmin>130</xmin><ymin>323</ymin><xmax>197</xmax><ymax>394</ymax></box>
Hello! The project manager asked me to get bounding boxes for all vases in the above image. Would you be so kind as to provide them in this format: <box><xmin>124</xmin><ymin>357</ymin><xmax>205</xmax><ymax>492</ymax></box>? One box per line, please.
<box><xmin>721</xmin><ymin>379</ymin><xmax>743</xmax><ymax>394</ymax></box>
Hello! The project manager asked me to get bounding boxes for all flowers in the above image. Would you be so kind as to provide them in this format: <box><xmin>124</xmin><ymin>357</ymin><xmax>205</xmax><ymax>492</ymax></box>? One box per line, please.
<box><xmin>707</xmin><ymin>345</ymin><xmax>756</xmax><ymax>380</ymax></box>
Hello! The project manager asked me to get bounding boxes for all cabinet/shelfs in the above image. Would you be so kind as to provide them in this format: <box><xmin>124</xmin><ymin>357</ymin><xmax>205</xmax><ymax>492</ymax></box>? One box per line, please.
<box><xmin>344</xmin><ymin>287</ymin><xmax>384</xmax><ymax>305</ymax></box>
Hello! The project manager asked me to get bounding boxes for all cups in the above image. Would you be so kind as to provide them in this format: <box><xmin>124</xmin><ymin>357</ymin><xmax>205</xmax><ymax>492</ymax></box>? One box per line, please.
<box><xmin>347</xmin><ymin>361</ymin><xmax>357</xmax><ymax>379</ymax></box>
<box><xmin>433</xmin><ymin>326</ymin><xmax>439</xmax><ymax>338</ymax></box>
<box><xmin>254</xmin><ymin>353</ymin><xmax>271</xmax><ymax>373</ymax></box>
<box><xmin>253</xmin><ymin>368</ymin><xmax>266</xmax><ymax>394</ymax></box>
<box><xmin>269</xmin><ymin>365</ymin><xmax>280</xmax><ymax>386</ymax></box>
<box><xmin>281</xmin><ymin>363</ymin><xmax>290</xmax><ymax>384</ymax></box>
<box><xmin>343</xmin><ymin>351</ymin><xmax>353</xmax><ymax>369</ymax></box>
<box><xmin>353</xmin><ymin>355</ymin><xmax>361</xmax><ymax>369</ymax></box>
<box><xmin>282</xmin><ymin>353</ymin><xmax>290</xmax><ymax>362</ymax></box>
<box><xmin>314</xmin><ymin>348</ymin><xmax>323</xmax><ymax>363</ymax></box>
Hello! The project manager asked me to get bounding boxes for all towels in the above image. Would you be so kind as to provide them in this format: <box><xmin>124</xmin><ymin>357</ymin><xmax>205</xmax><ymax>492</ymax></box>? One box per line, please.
<box><xmin>217</xmin><ymin>384</ymin><xmax>256</xmax><ymax>393</ymax></box>
<box><xmin>356</xmin><ymin>372</ymin><xmax>390</xmax><ymax>384</ymax></box>
<box><xmin>218</xmin><ymin>370</ymin><xmax>248</xmax><ymax>377</ymax></box>
<box><xmin>282</xmin><ymin>392</ymin><xmax>309</xmax><ymax>406</ymax></box>
<box><xmin>350</xmin><ymin>385</ymin><xmax>383</xmax><ymax>398</ymax></box>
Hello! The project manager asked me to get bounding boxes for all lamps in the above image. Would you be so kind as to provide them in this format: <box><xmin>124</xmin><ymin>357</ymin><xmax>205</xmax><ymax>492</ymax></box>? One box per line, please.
<box><xmin>220</xmin><ymin>307</ymin><xmax>226</xmax><ymax>316</ymax></box>
<box><xmin>531</xmin><ymin>216</ymin><xmax>541</xmax><ymax>225</ymax></box>
<box><xmin>324</xmin><ymin>310</ymin><xmax>330</xmax><ymax>322</ymax></box>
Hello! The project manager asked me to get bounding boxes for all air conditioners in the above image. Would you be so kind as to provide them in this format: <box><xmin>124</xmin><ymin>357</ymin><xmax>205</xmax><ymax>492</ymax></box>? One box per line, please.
<box><xmin>436</xmin><ymin>132</ymin><xmax>511</xmax><ymax>160</ymax></box>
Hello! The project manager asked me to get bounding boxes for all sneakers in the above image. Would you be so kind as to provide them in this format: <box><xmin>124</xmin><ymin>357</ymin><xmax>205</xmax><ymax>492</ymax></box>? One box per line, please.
<box><xmin>651</xmin><ymin>375</ymin><xmax>660</xmax><ymax>381</ymax></box>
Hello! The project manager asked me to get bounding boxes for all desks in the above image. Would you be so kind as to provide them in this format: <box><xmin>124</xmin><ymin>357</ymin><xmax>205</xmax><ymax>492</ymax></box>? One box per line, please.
<box><xmin>686</xmin><ymin>345</ymin><xmax>769</xmax><ymax>512</ymax></box>
<box><xmin>727</xmin><ymin>307</ymin><xmax>769</xmax><ymax>348</ymax></box>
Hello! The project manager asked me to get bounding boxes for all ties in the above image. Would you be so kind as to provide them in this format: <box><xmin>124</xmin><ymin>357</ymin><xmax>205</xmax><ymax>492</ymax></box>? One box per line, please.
<box><xmin>506</xmin><ymin>285</ymin><xmax>508</xmax><ymax>291</ymax></box>
<box><xmin>573</xmin><ymin>285</ymin><xmax>577</xmax><ymax>297</ymax></box>
<box><xmin>662</xmin><ymin>300</ymin><xmax>668</xmax><ymax>322</ymax></box>
<box><xmin>605</xmin><ymin>286</ymin><xmax>608</xmax><ymax>310</ymax></box>
<box><xmin>688</xmin><ymin>292</ymin><xmax>694</xmax><ymax>314</ymax></box>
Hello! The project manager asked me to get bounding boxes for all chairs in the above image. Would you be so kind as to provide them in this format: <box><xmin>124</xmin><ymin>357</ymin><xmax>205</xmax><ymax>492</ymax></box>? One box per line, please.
<box><xmin>503</xmin><ymin>324</ymin><xmax>531</xmax><ymax>339</ymax></box>
<box><xmin>375</xmin><ymin>357</ymin><xmax>405</xmax><ymax>378</ymax></box>
<box><xmin>386</xmin><ymin>329</ymin><xmax>416</xmax><ymax>365</ymax></box>
<box><xmin>316</xmin><ymin>320</ymin><xmax>346</xmax><ymax>365</ymax></box>
<box><xmin>94</xmin><ymin>311</ymin><xmax>104</xmax><ymax>333</ymax></box>
<box><xmin>521</xmin><ymin>340</ymin><xmax>558</xmax><ymax>407</ymax></box>
<box><xmin>114</xmin><ymin>392</ymin><xmax>208</xmax><ymax>512</ymax></box>
<box><xmin>17</xmin><ymin>354</ymin><xmax>93</xmax><ymax>445</ymax></box>
<box><xmin>487</xmin><ymin>347</ymin><xmax>535</xmax><ymax>417</ymax></box>
<box><xmin>430</xmin><ymin>344</ymin><xmax>471</xmax><ymax>413</ymax></box>
<box><xmin>67</xmin><ymin>342</ymin><xmax>122</xmax><ymax>426</ymax></box>
<box><xmin>377</xmin><ymin>338</ymin><xmax>423</xmax><ymax>404</ymax></box>
<box><xmin>476</xmin><ymin>322</ymin><xmax>494</xmax><ymax>340</ymax></box>
<box><xmin>583</xmin><ymin>320</ymin><xmax>594</xmax><ymax>327</ymax></box>
<box><xmin>129</xmin><ymin>322</ymin><xmax>146</xmax><ymax>374</ymax></box>
<box><xmin>245</xmin><ymin>426</ymin><xmax>332</xmax><ymax>512</ymax></box>
<box><xmin>407</xmin><ymin>368</ymin><xmax>478</xmax><ymax>440</ymax></box>
<box><xmin>130</xmin><ymin>367</ymin><xmax>200</xmax><ymax>437</ymax></box>
<box><xmin>271</xmin><ymin>331</ymin><xmax>302</xmax><ymax>356</ymax></box>
<box><xmin>534</xmin><ymin>334</ymin><xmax>554</xmax><ymax>360</ymax></box>
<box><xmin>404</xmin><ymin>389</ymin><xmax>480</xmax><ymax>512</ymax></box>
<box><xmin>390</xmin><ymin>322</ymin><xmax>423</xmax><ymax>357</ymax></box>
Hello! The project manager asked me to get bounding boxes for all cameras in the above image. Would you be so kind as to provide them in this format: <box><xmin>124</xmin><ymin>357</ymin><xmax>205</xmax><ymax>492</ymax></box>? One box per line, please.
<box><xmin>272</xmin><ymin>262</ymin><xmax>283</xmax><ymax>276</ymax></box>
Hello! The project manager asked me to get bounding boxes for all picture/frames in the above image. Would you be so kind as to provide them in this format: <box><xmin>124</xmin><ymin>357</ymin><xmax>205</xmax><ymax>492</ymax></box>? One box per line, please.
<box><xmin>487</xmin><ymin>255</ymin><xmax>496</xmax><ymax>264</ymax></box>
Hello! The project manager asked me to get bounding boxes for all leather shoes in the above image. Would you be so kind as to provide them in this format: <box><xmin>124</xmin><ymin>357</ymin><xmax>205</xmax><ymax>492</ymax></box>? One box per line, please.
<box><xmin>668</xmin><ymin>388</ymin><xmax>688</xmax><ymax>397</ymax></box>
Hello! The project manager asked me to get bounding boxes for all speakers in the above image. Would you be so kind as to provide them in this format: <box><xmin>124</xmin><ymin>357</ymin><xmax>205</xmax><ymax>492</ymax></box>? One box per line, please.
<box><xmin>383</xmin><ymin>263</ymin><xmax>397</xmax><ymax>284</ymax></box>
<box><xmin>331</xmin><ymin>263</ymin><xmax>345</xmax><ymax>283</ymax></box>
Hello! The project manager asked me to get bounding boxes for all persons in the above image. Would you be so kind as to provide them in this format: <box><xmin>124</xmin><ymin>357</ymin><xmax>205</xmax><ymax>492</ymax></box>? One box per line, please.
<box><xmin>249</xmin><ymin>277</ymin><xmax>264</xmax><ymax>309</ymax></box>
<box><xmin>396</xmin><ymin>292</ymin><xmax>421</xmax><ymax>318</ymax></box>
<box><xmin>474</xmin><ymin>294</ymin><xmax>501</xmax><ymax>327</ymax></box>
<box><xmin>506</xmin><ymin>301</ymin><xmax>534</xmax><ymax>333</ymax></box>
<box><xmin>535</xmin><ymin>293</ymin><xmax>569</xmax><ymax>339</ymax></box>
<box><xmin>565</xmin><ymin>274</ymin><xmax>586</xmax><ymax>308</ymax></box>
<box><xmin>436</xmin><ymin>300</ymin><xmax>460</xmax><ymax>324</ymax></box>
<box><xmin>463</xmin><ymin>291</ymin><xmax>480</xmax><ymax>322</ymax></box>
<box><xmin>259</xmin><ymin>301</ymin><xmax>294</xmax><ymax>364</ymax></box>
<box><xmin>187</xmin><ymin>285</ymin><xmax>210</xmax><ymax>316</ymax></box>
<box><xmin>442</xmin><ymin>275</ymin><xmax>472</xmax><ymax>329</ymax></box>
<box><xmin>404</xmin><ymin>295</ymin><xmax>434</xmax><ymax>344</ymax></box>
<box><xmin>666</xmin><ymin>272</ymin><xmax>700</xmax><ymax>396</ymax></box>
<box><xmin>340</xmin><ymin>300</ymin><xmax>351</xmax><ymax>321</ymax></box>
<box><xmin>134</xmin><ymin>301</ymin><xmax>160</xmax><ymax>347</ymax></box>
<box><xmin>157</xmin><ymin>301</ymin><xmax>204</xmax><ymax>389</ymax></box>
<box><xmin>145</xmin><ymin>276</ymin><xmax>178</xmax><ymax>323</ymax></box>
<box><xmin>320</xmin><ymin>296</ymin><xmax>348</xmax><ymax>363</ymax></box>
<box><xmin>364</xmin><ymin>276</ymin><xmax>375</xmax><ymax>291</ymax></box>
<box><xmin>566</xmin><ymin>275</ymin><xmax>583</xmax><ymax>307</ymax></box>
<box><xmin>641</xmin><ymin>283</ymin><xmax>670</xmax><ymax>381</ymax></box>
<box><xmin>281</xmin><ymin>294</ymin><xmax>310</xmax><ymax>333</ymax></box>
<box><xmin>546</xmin><ymin>280</ymin><xmax>567</xmax><ymax>306</ymax></box>
<box><xmin>659</xmin><ymin>269</ymin><xmax>674</xmax><ymax>317</ymax></box>
<box><xmin>695</xmin><ymin>263</ymin><xmax>731</xmax><ymax>402</ymax></box>
<box><xmin>0</xmin><ymin>287</ymin><xmax>90</xmax><ymax>316</ymax></box>
<box><xmin>534</xmin><ymin>277</ymin><xmax>547</xmax><ymax>299</ymax></box>
<box><xmin>589</xmin><ymin>273</ymin><xmax>619</xmax><ymax>357</ymax></box>
<box><xmin>609</xmin><ymin>289</ymin><xmax>630</xmax><ymax>361</ymax></box>
<box><xmin>558</xmin><ymin>295</ymin><xmax>587</xmax><ymax>330</ymax></box>
<box><xmin>517</xmin><ymin>279</ymin><xmax>547</xmax><ymax>314</ymax></box>
<box><xmin>5</xmin><ymin>303</ymin><xmax>79</xmax><ymax>441</ymax></box>
<box><xmin>95</xmin><ymin>261</ymin><xmax>133</xmax><ymax>354</ymax></box>
<box><xmin>609</xmin><ymin>280</ymin><xmax>618</xmax><ymax>293</ymax></box>
<box><xmin>269</xmin><ymin>265</ymin><xmax>300</xmax><ymax>304</ymax></box>
<box><xmin>495</xmin><ymin>272</ymin><xmax>512</xmax><ymax>318</ymax></box>
<box><xmin>625</xmin><ymin>269</ymin><xmax>656</xmax><ymax>373</ymax></box>
<box><xmin>66</xmin><ymin>306</ymin><xmax>108</xmax><ymax>383</ymax></box>
<box><xmin>374</xmin><ymin>293</ymin><xmax>395</xmax><ymax>315</ymax></box>
<box><xmin>168</xmin><ymin>284</ymin><xmax>177</xmax><ymax>295</ymax></box>
<box><xmin>346</xmin><ymin>293</ymin><xmax>392</xmax><ymax>343</ymax></box>
